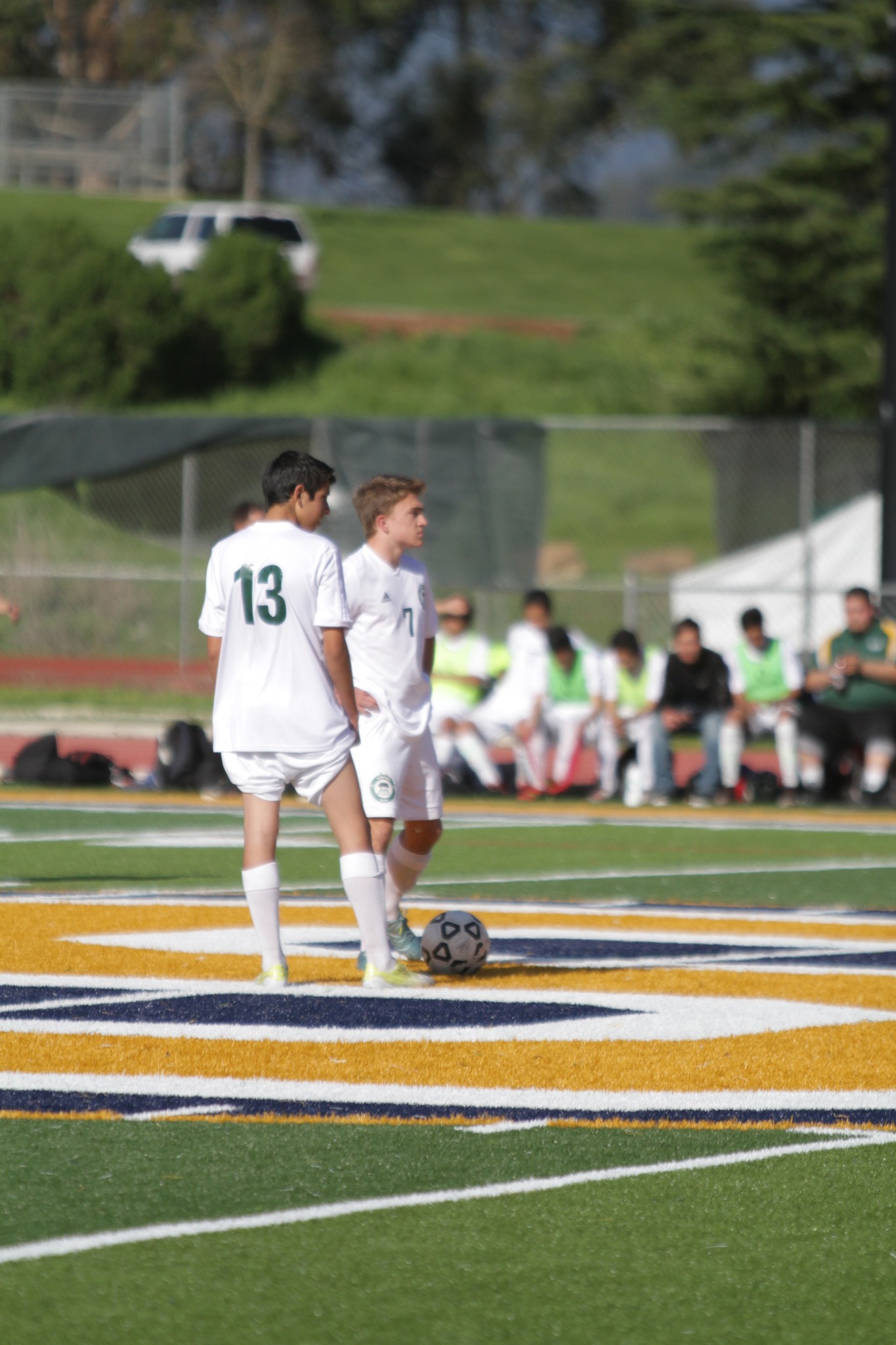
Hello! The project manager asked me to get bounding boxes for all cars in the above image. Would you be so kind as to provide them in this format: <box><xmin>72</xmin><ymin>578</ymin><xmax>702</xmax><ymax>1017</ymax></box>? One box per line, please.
<box><xmin>126</xmin><ymin>200</ymin><xmax>318</xmax><ymax>294</ymax></box>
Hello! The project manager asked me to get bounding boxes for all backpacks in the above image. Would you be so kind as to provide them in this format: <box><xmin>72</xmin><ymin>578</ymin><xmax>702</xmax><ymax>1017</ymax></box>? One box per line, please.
<box><xmin>160</xmin><ymin>723</ymin><xmax>211</xmax><ymax>784</ymax></box>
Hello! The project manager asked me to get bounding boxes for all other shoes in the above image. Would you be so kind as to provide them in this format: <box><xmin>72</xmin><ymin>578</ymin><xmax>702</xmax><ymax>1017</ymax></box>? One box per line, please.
<box><xmin>651</xmin><ymin>789</ymin><xmax>669</xmax><ymax>807</ymax></box>
<box><xmin>688</xmin><ymin>793</ymin><xmax>713</xmax><ymax>808</ymax></box>
<box><xmin>592</xmin><ymin>789</ymin><xmax>613</xmax><ymax>802</ymax></box>
<box><xmin>517</xmin><ymin>784</ymin><xmax>544</xmax><ymax>802</ymax></box>
<box><xmin>779</xmin><ymin>787</ymin><xmax>798</xmax><ymax>807</ymax></box>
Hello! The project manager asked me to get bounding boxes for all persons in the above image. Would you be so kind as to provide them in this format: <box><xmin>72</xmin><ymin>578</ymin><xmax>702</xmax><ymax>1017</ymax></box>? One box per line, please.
<box><xmin>199</xmin><ymin>450</ymin><xmax>434</xmax><ymax>988</ymax></box>
<box><xmin>423</xmin><ymin>588</ymin><xmax>896</xmax><ymax>809</ymax></box>
<box><xmin>0</xmin><ymin>596</ymin><xmax>19</xmax><ymax>620</ymax></box>
<box><xmin>201</xmin><ymin>501</ymin><xmax>266</xmax><ymax>803</ymax></box>
<box><xmin>341</xmin><ymin>475</ymin><xmax>443</xmax><ymax>964</ymax></box>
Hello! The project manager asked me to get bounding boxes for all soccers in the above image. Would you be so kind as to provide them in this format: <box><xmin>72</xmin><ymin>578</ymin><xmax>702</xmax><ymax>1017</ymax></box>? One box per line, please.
<box><xmin>419</xmin><ymin>909</ymin><xmax>490</xmax><ymax>978</ymax></box>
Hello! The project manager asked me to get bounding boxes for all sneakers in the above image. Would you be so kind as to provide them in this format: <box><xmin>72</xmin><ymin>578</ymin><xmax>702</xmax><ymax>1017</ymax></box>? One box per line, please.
<box><xmin>356</xmin><ymin>951</ymin><xmax>369</xmax><ymax>970</ymax></box>
<box><xmin>363</xmin><ymin>963</ymin><xmax>435</xmax><ymax>990</ymax></box>
<box><xmin>256</xmin><ymin>963</ymin><xmax>288</xmax><ymax>988</ymax></box>
<box><xmin>387</xmin><ymin>914</ymin><xmax>424</xmax><ymax>961</ymax></box>
